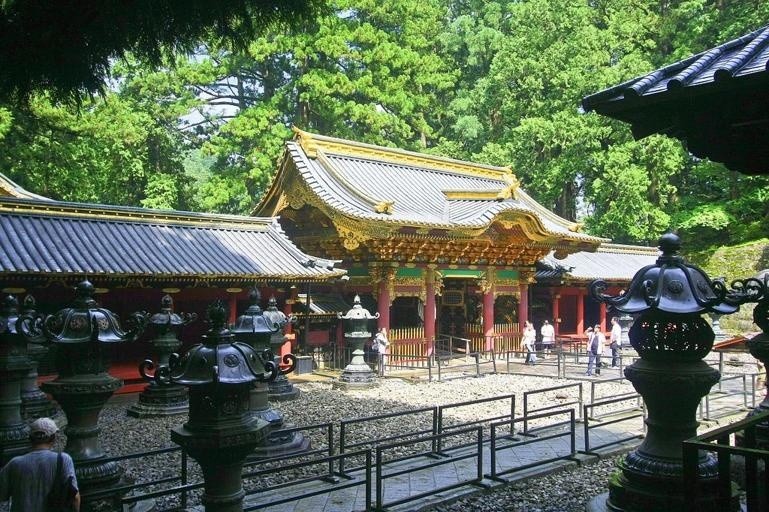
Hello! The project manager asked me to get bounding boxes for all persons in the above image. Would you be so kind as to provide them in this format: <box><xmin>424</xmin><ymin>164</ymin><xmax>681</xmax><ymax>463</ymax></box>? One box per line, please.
<box><xmin>521</xmin><ymin>323</ymin><xmax>536</xmax><ymax>365</ymax></box>
<box><xmin>0</xmin><ymin>417</ymin><xmax>81</xmax><ymax>512</ymax></box>
<box><xmin>372</xmin><ymin>328</ymin><xmax>389</xmax><ymax>375</ymax></box>
<box><xmin>540</xmin><ymin>319</ymin><xmax>555</xmax><ymax>359</ymax></box>
<box><xmin>610</xmin><ymin>316</ymin><xmax>622</xmax><ymax>369</ymax></box>
<box><xmin>523</xmin><ymin>321</ymin><xmax>530</xmax><ymax>333</ymax></box>
<box><xmin>584</xmin><ymin>324</ymin><xmax>606</xmax><ymax>377</ymax></box>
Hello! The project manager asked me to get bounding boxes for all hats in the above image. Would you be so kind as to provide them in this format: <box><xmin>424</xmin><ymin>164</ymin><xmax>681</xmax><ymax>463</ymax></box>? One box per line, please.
<box><xmin>30</xmin><ymin>418</ymin><xmax>59</xmax><ymax>438</ymax></box>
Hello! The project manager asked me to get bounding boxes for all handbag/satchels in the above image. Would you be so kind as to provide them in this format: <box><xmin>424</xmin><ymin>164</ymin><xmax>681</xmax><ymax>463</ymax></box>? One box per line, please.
<box><xmin>47</xmin><ymin>476</ymin><xmax>77</xmax><ymax>512</ymax></box>
